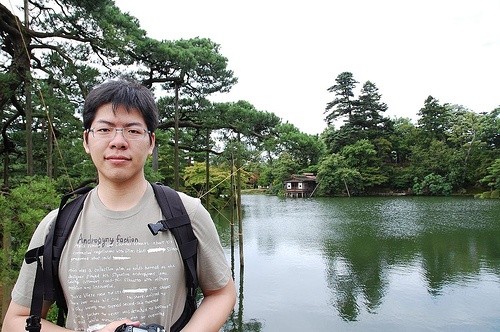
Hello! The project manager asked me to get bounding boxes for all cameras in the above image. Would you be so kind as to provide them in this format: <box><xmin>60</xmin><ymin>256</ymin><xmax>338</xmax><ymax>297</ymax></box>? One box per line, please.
<box><xmin>115</xmin><ymin>324</ymin><xmax>167</xmax><ymax>332</ymax></box>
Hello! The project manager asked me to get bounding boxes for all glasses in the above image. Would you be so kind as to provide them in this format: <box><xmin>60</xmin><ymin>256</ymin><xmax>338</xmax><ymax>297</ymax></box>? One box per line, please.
<box><xmin>86</xmin><ymin>127</ymin><xmax>151</xmax><ymax>140</ymax></box>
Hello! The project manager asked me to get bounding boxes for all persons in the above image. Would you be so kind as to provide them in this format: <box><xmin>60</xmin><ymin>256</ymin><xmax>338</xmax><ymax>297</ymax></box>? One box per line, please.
<box><xmin>0</xmin><ymin>82</ymin><xmax>237</xmax><ymax>332</ymax></box>
